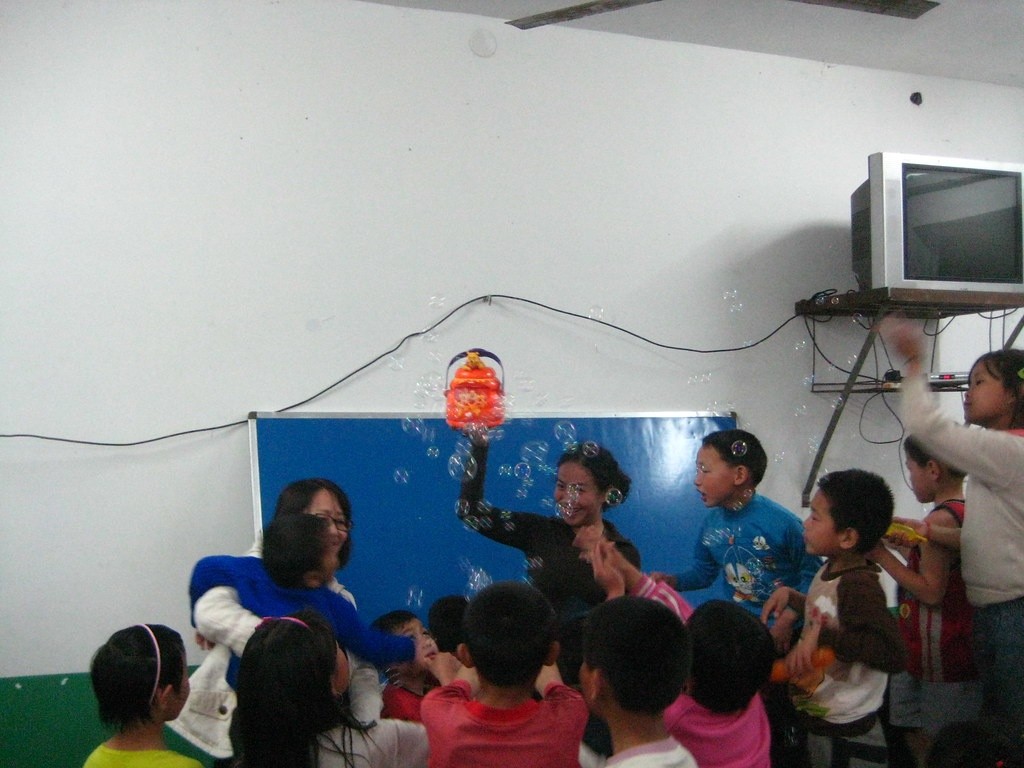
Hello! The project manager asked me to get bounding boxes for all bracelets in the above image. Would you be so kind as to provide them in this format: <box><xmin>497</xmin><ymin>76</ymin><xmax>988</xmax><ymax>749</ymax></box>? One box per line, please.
<box><xmin>900</xmin><ymin>351</ymin><xmax>923</xmax><ymax>367</ymax></box>
<box><xmin>924</xmin><ymin>520</ymin><xmax>932</xmax><ymax>539</ymax></box>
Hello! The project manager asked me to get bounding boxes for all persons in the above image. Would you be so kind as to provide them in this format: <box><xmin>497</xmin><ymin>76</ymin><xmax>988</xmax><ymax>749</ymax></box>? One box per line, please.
<box><xmin>578</xmin><ymin>595</ymin><xmax>700</xmax><ymax>768</ymax></box>
<box><xmin>451</xmin><ymin>421</ymin><xmax>644</xmax><ymax>759</ymax></box>
<box><xmin>420</xmin><ymin>579</ymin><xmax>590</xmax><ymax>768</ymax></box>
<box><xmin>864</xmin><ymin>317</ymin><xmax>1024</xmax><ymax>768</ymax></box>
<box><xmin>588</xmin><ymin>538</ymin><xmax>777</xmax><ymax>768</ymax></box>
<box><xmin>82</xmin><ymin>623</ymin><xmax>204</xmax><ymax>768</ymax></box>
<box><xmin>157</xmin><ymin>479</ymin><xmax>470</xmax><ymax>768</ymax></box>
<box><xmin>650</xmin><ymin>427</ymin><xmax>909</xmax><ymax>768</ymax></box>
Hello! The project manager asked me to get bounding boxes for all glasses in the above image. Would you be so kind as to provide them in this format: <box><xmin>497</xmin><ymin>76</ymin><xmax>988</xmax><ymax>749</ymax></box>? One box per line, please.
<box><xmin>301</xmin><ymin>509</ymin><xmax>354</xmax><ymax>533</ymax></box>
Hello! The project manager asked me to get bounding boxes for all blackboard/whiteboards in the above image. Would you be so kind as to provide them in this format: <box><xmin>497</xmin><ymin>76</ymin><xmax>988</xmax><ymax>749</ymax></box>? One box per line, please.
<box><xmin>249</xmin><ymin>410</ymin><xmax>739</xmax><ymax>686</ymax></box>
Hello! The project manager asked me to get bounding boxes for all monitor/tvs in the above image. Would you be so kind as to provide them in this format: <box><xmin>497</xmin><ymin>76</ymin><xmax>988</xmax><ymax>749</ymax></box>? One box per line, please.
<box><xmin>850</xmin><ymin>151</ymin><xmax>1024</xmax><ymax>293</ymax></box>
